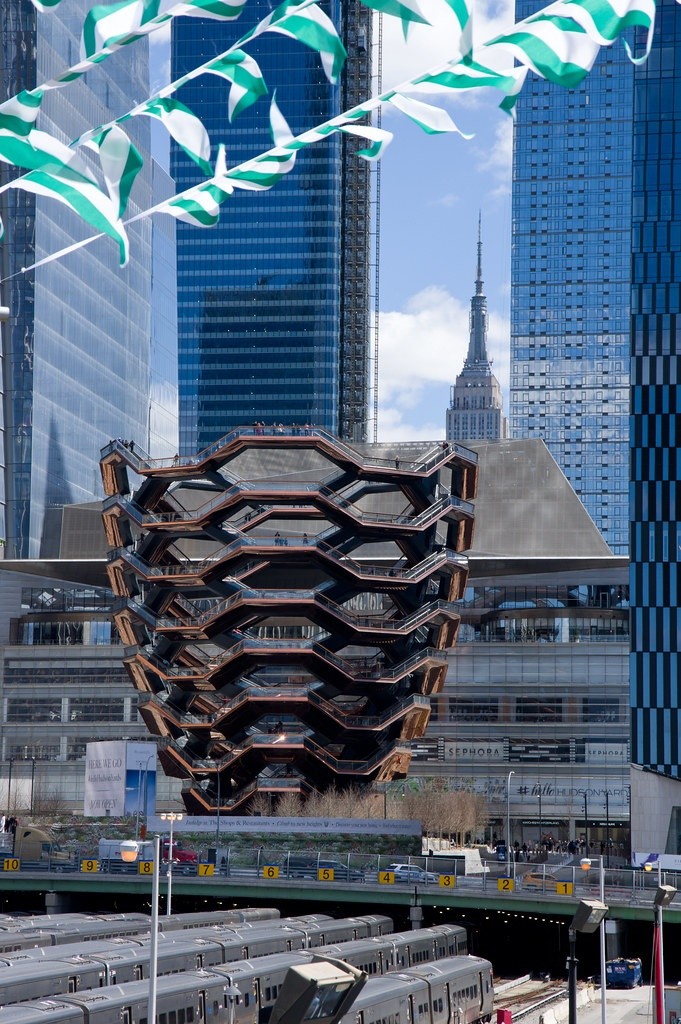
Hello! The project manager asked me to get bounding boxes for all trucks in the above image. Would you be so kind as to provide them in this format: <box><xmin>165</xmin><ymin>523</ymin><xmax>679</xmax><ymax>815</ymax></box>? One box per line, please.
<box><xmin>98</xmin><ymin>838</ymin><xmax>199</xmax><ymax>876</ymax></box>
<box><xmin>0</xmin><ymin>827</ymin><xmax>75</xmax><ymax>874</ymax></box>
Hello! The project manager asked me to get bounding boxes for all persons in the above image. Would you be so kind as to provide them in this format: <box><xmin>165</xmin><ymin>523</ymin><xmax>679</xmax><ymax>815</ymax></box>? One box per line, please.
<box><xmin>442</xmin><ymin>441</ymin><xmax>458</xmax><ymax>457</ymax></box>
<box><xmin>109</xmin><ymin>438</ymin><xmax>134</xmax><ymax>452</ymax></box>
<box><xmin>0</xmin><ymin>814</ymin><xmax>19</xmax><ymax>833</ymax></box>
<box><xmin>542</xmin><ymin>836</ymin><xmax>586</xmax><ymax>854</ymax></box>
<box><xmin>514</xmin><ymin>840</ymin><xmax>527</xmax><ymax>852</ymax></box>
<box><xmin>174</xmin><ymin>453</ymin><xmax>178</xmax><ymax>467</ymax></box>
<box><xmin>273</xmin><ymin>532</ymin><xmax>308</xmax><ymax>547</ymax></box>
<box><xmin>251</xmin><ymin>420</ymin><xmax>313</xmax><ymax>436</ymax></box>
<box><xmin>395</xmin><ymin>456</ymin><xmax>400</xmax><ymax>469</ymax></box>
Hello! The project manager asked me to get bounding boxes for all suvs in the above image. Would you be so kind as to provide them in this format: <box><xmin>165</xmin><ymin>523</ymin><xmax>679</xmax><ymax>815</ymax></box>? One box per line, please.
<box><xmin>386</xmin><ymin>864</ymin><xmax>439</xmax><ymax>884</ymax></box>
<box><xmin>307</xmin><ymin>860</ymin><xmax>360</xmax><ymax>882</ymax></box>
<box><xmin>522</xmin><ymin>873</ymin><xmax>558</xmax><ymax>893</ymax></box>
<box><xmin>283</xmin><ymin>857</ymin><xmax>316</xmax><ymax>879</ymax></box>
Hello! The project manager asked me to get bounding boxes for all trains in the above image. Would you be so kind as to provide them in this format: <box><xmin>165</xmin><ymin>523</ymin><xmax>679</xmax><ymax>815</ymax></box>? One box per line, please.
<box><xmin>0</xmin><ymin>908</ymin><xmax>280</xmax><ymax>952</ymax></box>
<box><xmin>338</xmin><ymin>953</ymin><xmax>494</xmax><ymax>1024</ymax></box>
<box><xmin>0</xmin><ymin>924</ymin><xmax>467</xmax><ymax>1024</ymax></box>
<box><xmin>0</xmin><ymin>915</ymin><xmax>394</xmax><ymax>1005</ymax></box>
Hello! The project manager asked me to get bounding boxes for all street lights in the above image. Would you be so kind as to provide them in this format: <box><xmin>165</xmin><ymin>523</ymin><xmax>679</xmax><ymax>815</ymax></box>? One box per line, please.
<box><xmin>120</xmin><ymin>834</ymin><xmax>159</xmax><ymax>1024</ymax></box>
<box><xmin>653</xmin><ymin>885</ymin><xmax>676</xmax><ymax>1024</ymax></box>
<box><xmin>160</xmin><ymin>811</ymin><xmax>182</xmax><ymax>915</ymax></box>
<box><xmin>505</xmin><ymin>770</ymin><xmax>516</xmax><ymax>877</ymax></box>
<box><xmin>645</xmin><ymin>860</ymin><xmax>666</xmax><ymax>1024</ymax></box>
<box><xmin>144</xmin><ymin>754</ymin><xmax>154</xmax><ymax>840</ymax></box>
<box><xmin>580</xmin><ymin>855</ymin><xmax>606</xmax><ymax>1024</ymax></box>
<box><xmin>567</xmin><ymin>899</ymin><xmax>608</xmax><ymax>1024</ymax></box>
